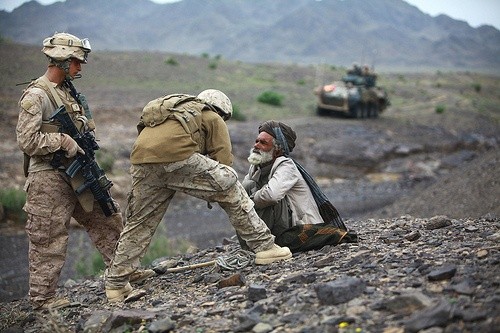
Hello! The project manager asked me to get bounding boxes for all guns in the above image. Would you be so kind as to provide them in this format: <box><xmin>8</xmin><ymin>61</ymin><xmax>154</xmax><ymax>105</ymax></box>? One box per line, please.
<box><xmin>48</xmin><ymin>105</ymin><xmax>118</xmax><ymax>217</ymax></box>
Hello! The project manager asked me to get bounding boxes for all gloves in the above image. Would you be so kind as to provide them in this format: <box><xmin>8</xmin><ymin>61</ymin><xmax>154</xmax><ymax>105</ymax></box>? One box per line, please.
<box><xmin>60</xmin><ymin>133</ymin><xmax>86</xmax><ymax>159</ymax></box>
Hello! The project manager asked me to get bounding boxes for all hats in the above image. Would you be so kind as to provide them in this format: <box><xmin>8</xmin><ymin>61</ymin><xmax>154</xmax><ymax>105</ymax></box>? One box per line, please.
<box><xmin>194</xmin><ymin>88</ymin><xmax>233</xmax><ymax>122</ymax></box>
<box><xmin>41</xmin><ymin>32</ymin><xmax>87</xmax><ymax>62</ymax></box>
<box><xmin>258</xmin><ymin>120</ymin><xmax>297</xmax><ymax>153</ymax></box>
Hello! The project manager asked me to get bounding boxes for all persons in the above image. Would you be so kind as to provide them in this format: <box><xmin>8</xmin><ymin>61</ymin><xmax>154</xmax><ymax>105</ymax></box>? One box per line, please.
<box><xmin>242</xmin><ymin>121</ymin><xmax>324</xmax><ymax>240</ymax></box>
<box><xmin>16</xmin><ymin>32</ymin><xmax>155</xmax><ymax>311</ymax></box>
<box><xmin>349</xmin><ymin>64</ymin><xmax>370</xmax><ymax>76</ymax></box>
<box><xmin>105</xmin><ymin>89</ymin><xmax>292</xmax><ymax>302</ymax></box>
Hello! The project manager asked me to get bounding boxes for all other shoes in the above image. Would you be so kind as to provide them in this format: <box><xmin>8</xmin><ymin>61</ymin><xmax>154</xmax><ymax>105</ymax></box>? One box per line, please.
<box><xmin>33</xmin><ymin>298</ymin><xmax>70</xmax><ymax>312</ymax></box>
<box><xmin>105</xmin><ymin>280</ymin><xmax>133</xmax><ymax>302</ymax></box>
<box><xmin>129</xmin><ymin>268</ymin><xmax>155</xmax><ymax>284</ymax></box>
<box><xmin>253</xmin><ymin>241</ymin><xmax>292</xmax><ymax>264</ymax></box>
